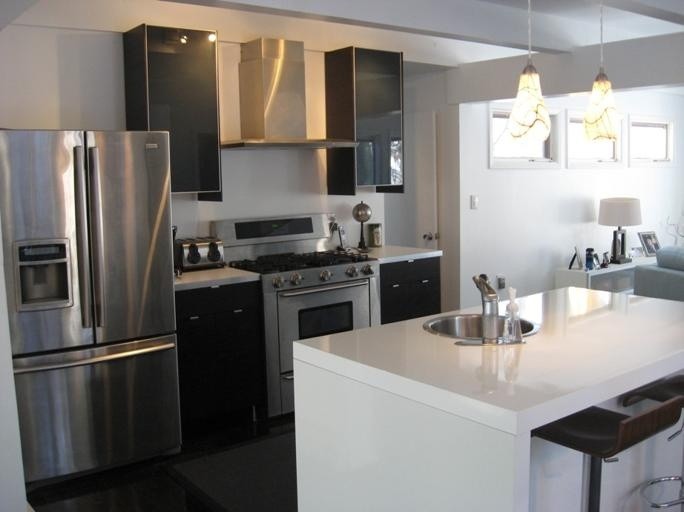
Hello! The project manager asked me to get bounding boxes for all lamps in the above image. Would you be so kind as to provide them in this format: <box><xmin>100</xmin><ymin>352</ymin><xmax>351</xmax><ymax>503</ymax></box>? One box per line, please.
<box><xmin>583</xmin><ymin>1</ymin><xmax>618</xmax><ymax>142</ymax></box>
<box><xmin>508</xmin><ymin>1</ymin><xmax>552</xmax><ymax>145</ymax></box>
<box><xmin>598</xmin><ymin>198</ymin><xmax>642</xmax><ymax>265</ymax></box>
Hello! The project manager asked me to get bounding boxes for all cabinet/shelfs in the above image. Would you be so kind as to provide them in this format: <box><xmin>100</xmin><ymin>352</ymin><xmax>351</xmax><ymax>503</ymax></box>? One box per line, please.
<box><xmin>174</xmin><ymin>274</ymin><xmax>270</xmax><ymax>453</ymax></box>
<box><xmin>380</xmin><ymin>250</ymin><xmax>441</xmax><ymax>325</ymax></box>
<box><xmin>324</xmin><ymin>46</ymin><xmax>406</xmax><ymax>196</ymax></box>
<box><xmin>123</xmin><ymin>23</ymin><xmax>220</xmax><ymax>194</ymax></box>
<box><xmin>555</xmin><ymin>256</ymin><xmax>658</xmax><ymax>292</ymax></box>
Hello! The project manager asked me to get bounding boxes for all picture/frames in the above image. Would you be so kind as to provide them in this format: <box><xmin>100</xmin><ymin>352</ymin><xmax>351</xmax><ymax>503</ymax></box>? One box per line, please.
<box><xmin>573</xmin><ymin>245</ymin><xmax>583</xmax><ymax>270</ymax></box>
<box><xmin>639</xmin><ymin>232</ymin><xmax>661</xmax><ymax>257</ymax></box>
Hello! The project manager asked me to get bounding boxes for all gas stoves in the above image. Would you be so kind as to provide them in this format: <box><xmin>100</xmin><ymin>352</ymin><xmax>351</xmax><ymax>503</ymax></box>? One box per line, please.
<box><xmin>212</xmin><ymin>212</ymin><xmax>383</xmax><ymax>293</ymax></box>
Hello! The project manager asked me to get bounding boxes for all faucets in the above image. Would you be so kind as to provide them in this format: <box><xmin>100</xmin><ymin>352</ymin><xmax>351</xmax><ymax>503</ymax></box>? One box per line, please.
<box><xmin>456</xmin><ymin>275</ymin><xmax>526</xmax><ymax>347</ymax></box>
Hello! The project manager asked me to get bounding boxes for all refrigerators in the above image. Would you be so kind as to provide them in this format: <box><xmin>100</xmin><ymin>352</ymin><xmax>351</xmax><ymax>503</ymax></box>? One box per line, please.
<box><xmin>3</xmin><ymin>128</ymin><xmax>183</xmax><ymax>484</ymax></box>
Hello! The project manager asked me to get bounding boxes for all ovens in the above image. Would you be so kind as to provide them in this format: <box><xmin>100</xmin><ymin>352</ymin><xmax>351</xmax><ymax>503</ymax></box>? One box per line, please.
<box><xmin>260</xmin><ymin>277</ymin><xmax>395</xmax><ymax>418</ymax></box>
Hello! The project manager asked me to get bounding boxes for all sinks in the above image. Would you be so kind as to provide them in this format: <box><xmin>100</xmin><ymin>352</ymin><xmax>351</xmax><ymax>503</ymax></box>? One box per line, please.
<box><xmin>423</xmin><ymin>312</ymin><xmax>539</xmax><ymax>340</ymax></box>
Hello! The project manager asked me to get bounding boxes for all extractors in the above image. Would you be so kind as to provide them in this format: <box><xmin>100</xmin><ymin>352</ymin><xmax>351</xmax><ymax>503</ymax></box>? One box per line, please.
<box><xmin>223</xmin><ymin>38</ymin><xmax>358</xmax><ymax>152</ymax></box>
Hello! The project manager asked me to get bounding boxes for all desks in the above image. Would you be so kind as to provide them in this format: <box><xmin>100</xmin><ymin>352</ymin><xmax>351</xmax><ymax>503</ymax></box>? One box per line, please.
<box><xmin>293</xmin><ymin>286</ymin><xmax>684</xmax><ymax>511</ymax></box>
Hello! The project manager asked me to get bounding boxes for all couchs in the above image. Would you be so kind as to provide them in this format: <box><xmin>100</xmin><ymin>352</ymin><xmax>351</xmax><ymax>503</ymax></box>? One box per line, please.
<box><xmin>634</xmin><ymin>263</ymin><xmax>684</xmax><ymax>302</ymax></box>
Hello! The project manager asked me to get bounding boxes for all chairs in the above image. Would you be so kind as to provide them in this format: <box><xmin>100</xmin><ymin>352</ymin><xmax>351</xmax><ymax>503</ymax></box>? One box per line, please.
<box><xmin>642</xmin><ymin>372</ymin><xmax>684</xmax><ymax>511</ymax></box>
<box><xmin>530</xmin><ymin>394</ymin><xmax>684</xmax><ymax>511</ymax></box>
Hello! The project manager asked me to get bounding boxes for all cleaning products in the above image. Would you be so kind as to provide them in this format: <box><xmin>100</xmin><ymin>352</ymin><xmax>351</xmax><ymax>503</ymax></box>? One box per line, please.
<box><xmin>500</xmin><ymin>286</ymin><xmax>522</xmax><ymax>343</ymax></box>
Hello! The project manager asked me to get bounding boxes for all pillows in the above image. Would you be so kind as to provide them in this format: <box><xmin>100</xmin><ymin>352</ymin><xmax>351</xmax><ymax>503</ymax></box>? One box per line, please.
<box><xmin>656</xmin><ymin>248</ymin><xmax>684</xmax><ymax>270</ymax></box>
